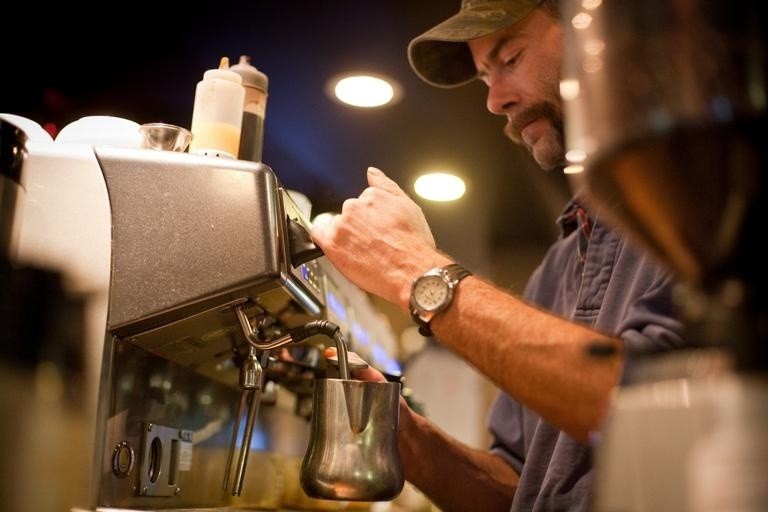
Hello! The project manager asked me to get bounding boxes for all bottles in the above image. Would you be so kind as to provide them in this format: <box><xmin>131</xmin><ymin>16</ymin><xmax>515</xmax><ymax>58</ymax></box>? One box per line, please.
<box><xmin>188</xmin><ymin>56</ymin><xmax>269</xmax><ymax>163</ymax></box>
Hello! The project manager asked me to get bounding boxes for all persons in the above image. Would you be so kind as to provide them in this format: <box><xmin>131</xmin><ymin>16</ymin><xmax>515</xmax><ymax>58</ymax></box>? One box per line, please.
<box><xmin>279</xmin><ymin>1</ymin><xmax>707</xmax><ymax>512</ymax></box>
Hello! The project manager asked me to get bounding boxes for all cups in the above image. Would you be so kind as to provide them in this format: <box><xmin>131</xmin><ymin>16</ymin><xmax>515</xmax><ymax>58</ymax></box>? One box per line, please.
<box><xmin>301</xmin><ymin>379</ymin><xmax>405</xmax><ymax>501</ymax></box>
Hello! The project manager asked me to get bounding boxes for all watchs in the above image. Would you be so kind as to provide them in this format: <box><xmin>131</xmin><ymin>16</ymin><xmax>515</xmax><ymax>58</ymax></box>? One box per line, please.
<box><xmin>408</xmin><ymin>263</ymin><xmax>473</xmax><ymax>337</ymax></box>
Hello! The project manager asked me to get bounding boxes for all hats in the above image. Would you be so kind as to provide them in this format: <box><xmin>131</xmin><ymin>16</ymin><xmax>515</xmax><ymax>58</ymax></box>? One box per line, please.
<box><xmin>407</xmin><ymin>1</ymin><xmax>548</xmax><ymax>92</ymax></box>
